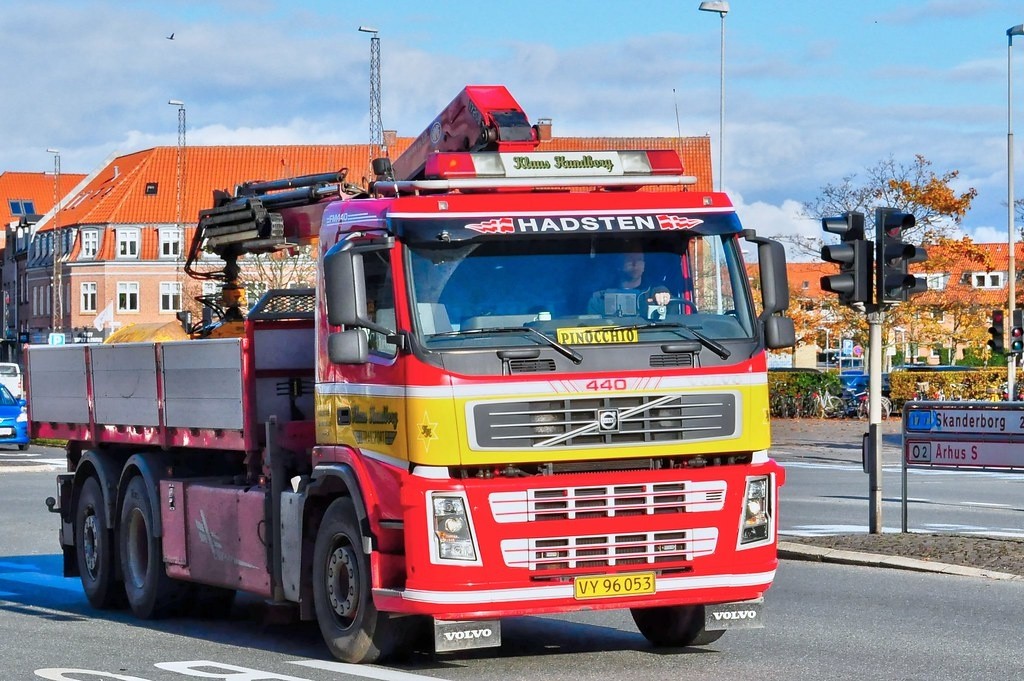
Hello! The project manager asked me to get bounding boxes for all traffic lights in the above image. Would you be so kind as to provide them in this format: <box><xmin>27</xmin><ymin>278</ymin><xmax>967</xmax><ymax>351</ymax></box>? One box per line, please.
<box><xmin>987</xmin><ymin>310</ymin><xmax>1007</xmax><ymax>352</ymax></box>
<box><xmin>876</xmin><ymin>206</ymin><xmax>916</xmax><ymax>307</ymax></box>
<box><xmin>819</xmin><ymin>210</ymin><xmax>869</xmax><ymax>306</ymax></box>
<box><xmin>1011</xmin><ymin>326</ymin><xmax>1023</xmax><ymax>355</ymax></box>
<box><xmin>907</xmin><ymin>244</ymin><xmax>930</xmax><ymax>299</ymax></box>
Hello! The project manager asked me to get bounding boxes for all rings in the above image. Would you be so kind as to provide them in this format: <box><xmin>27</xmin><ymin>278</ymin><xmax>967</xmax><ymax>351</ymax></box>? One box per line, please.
<box><xmin>663</xmin><ymin>296</ymin><xmax>668</xmax><ymax>299</ymax></box>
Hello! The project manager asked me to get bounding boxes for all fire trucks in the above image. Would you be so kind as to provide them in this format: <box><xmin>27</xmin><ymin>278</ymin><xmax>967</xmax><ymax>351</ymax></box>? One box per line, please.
<box><xmin>22</xmin><ymin>84</ymin><xmax>800</xmax><ymax>667</ymax></box>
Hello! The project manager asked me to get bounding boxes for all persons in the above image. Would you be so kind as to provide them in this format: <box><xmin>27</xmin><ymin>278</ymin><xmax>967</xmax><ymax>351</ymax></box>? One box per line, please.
<box><xmin>587</xmin><ymin>251</ymin><xmax>675</xmax><ymax>315</ymax></box>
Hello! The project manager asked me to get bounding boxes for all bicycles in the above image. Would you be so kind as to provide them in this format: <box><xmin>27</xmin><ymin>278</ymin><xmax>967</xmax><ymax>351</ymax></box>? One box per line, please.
<box><xmin>770</xmin><ymin>382</ymin><xmax>893</xmax><ymax>421</ymax></box>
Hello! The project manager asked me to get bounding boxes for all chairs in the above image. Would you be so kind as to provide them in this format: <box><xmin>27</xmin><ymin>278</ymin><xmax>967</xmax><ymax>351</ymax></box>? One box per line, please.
<box><xmin>568</xmin><ymin>258</ymin><xmax>624</xmax><ymax>315</ymax></box>
<box><xmin>460</xmin><ymin>266</ymin><xmax>529</xmax><ymax>322</ymax></box>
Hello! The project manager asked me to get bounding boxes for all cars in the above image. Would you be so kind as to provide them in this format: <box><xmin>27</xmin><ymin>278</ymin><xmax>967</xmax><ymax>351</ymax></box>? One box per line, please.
<box><xmin>847</xmin><ymin>373</ymin><xmax>893</xmax><ymax>418</ymax></box>
<box><xmin>838</xmin><ymin>369</ymin><xmax>863</xmax><ymax>390</ymax></box>
<box><xmin>0</xmin><ymin>383</ymin><xmax>31</xmax><ymax>451</ymax></box>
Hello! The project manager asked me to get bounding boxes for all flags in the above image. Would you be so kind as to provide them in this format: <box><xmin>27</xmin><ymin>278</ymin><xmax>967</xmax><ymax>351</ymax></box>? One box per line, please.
<box><xmin>93</xmin><ymin>303</ymin><xmax>113</xmax><ymax>332</ymax></box>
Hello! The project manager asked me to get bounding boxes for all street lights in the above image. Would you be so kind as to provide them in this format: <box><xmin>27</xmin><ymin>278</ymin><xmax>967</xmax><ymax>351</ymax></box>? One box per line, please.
<box><xmin>168</xmin><ymin>99</ymin><xmax>186</xmax><ymax>321</ymax></box>
<box><xmin>699</xmin><ymin>1</ymin><xmax>731</xmax><ymax>194</ymax></box>
<box><xmin>357</xmin><ymin>26</ymin><xmax>388</xmax><ymax>184</ymax></box>
<box><xmin>1003</xmin><ymin>22</ymin><xmax>1023</xmax><ymax>409</ymax></box>
<box><xmin>47</xmin><ymin>147</ymin><xmax>66</xmax><ymax>332</ymax></box>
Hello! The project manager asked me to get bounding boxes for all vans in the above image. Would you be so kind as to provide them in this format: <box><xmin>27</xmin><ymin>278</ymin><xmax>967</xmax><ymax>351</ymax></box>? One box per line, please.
<box><xmin>0</xmin><ymin>361</ymin><xmax>26</xmax><ymax>402</ymax></box>
